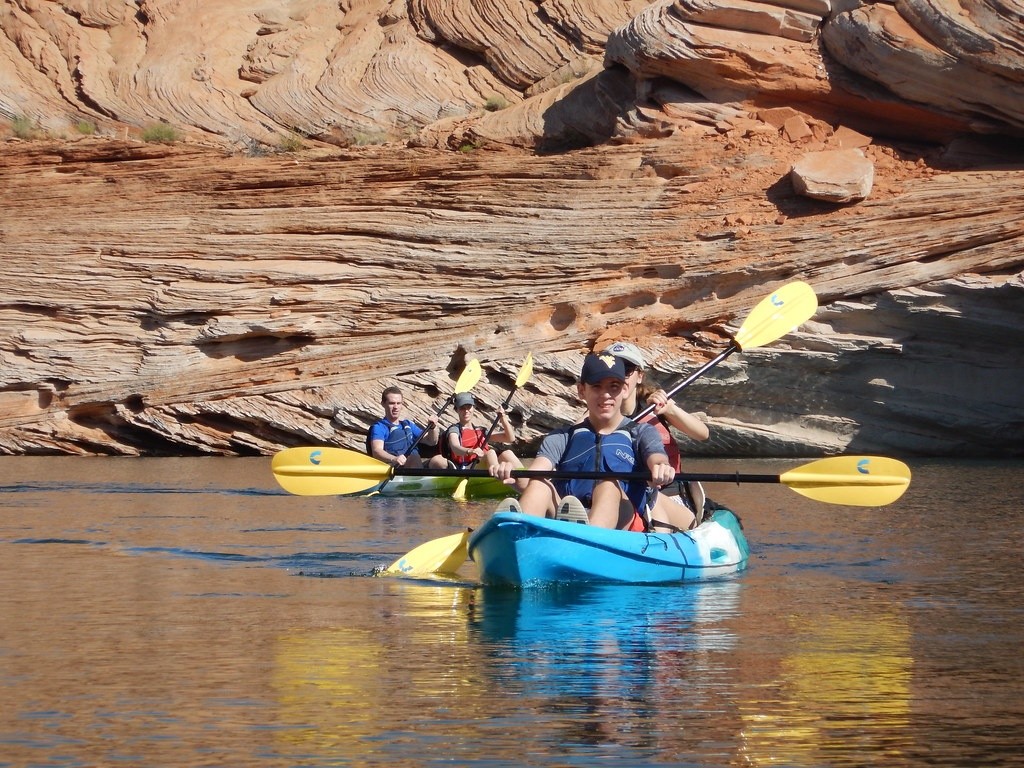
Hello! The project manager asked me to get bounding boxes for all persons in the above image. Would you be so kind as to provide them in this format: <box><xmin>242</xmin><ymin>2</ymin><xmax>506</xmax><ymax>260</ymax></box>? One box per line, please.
<box><xmin>605</xmin><ymin>343</ymin><xmax>708</xmax><ymax>509</ymax></box>
<box><xmin>488</xmin><ymin>353</ymin><xmax>675</xmax><ymax>533</ymax></box>
<box><xmin>446</xmin><ymin>392</ymin><xmax>525</xmax><ymax>467</ymax></box>
<box><xmin>371</xmin><ymin>387</ymin><xmax>448</xmax><ymax>469</ymax></box>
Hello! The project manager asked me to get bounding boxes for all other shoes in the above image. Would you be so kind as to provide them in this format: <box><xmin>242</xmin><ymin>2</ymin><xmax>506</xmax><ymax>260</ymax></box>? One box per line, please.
<box><xmin>556</xmin><ymin>495</ymin><xmax>589</xmax><ymax>525</ymax></box>
<box><xmin>493</xmin><ymin>498</ymin><xmax>523</xmax><ymax>514</ymax></box>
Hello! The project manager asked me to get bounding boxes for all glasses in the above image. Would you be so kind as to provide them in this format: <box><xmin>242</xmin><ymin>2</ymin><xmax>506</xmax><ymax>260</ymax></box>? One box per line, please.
<box><xmin>625</xmin><ymin>365</ymin><xmax>636</xmax><ymax>376</ymax></box>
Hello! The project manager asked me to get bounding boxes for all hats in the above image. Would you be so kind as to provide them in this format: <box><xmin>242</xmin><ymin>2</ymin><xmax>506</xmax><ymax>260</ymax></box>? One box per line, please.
<box><xmin>454</xmin><ymin>392</ymin><xmax>474</xmax><ymax>408</ymax></box>
<box><xmin>581</xmin><ymin>352</ymin><xmax>626</xmax><ymax>385</ymax></box>
<box><xmin>605</xmin><ymin>342</ymin><xmax>645</xmax><ymax>371</ymax></box>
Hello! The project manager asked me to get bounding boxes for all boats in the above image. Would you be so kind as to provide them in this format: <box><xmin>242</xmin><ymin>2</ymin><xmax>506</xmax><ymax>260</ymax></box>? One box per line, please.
<box><xmin>364</xmin><ymin>468</ymin><xmax>528</xmax><ymax>496</ymax></box>
<box><xmin>465</xmin><ymin>479</ymin><xmax>748</xmax><ymax>590</ymax></box>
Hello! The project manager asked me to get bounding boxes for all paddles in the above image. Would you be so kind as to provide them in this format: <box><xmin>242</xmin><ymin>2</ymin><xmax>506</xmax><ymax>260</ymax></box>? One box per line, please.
<box><xmin>451</xmin><ymin>350</ymin><xmax>534</xmax><ymax>501</ymax></box>
<box><xmin>380</xmin><ymin>281</ymin><xmax>818</xmax><ymax>576</ymax></box>
<box><xmin>365</xmin><ymin>359</ymin><xmax>482</xmax><ymax>499</ymax></box>
<box><xmin>270</xmin><ymin>446</ymin><xmax>914</xmax><ymax>508</ymax></box>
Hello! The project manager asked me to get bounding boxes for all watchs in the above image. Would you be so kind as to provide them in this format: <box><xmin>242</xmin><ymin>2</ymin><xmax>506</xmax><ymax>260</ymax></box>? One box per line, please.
<box><xmin>466</xmin><ymin>448</ymin><xmax>470</xmax><ymax>456</ymax></box>
